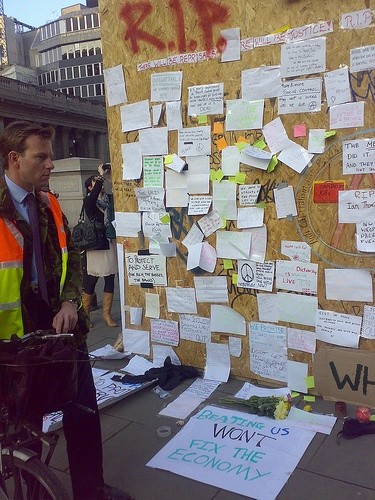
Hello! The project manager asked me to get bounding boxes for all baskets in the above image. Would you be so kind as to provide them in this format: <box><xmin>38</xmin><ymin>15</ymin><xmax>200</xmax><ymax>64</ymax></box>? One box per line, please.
<box><xmin>0</xmin><ymin>349</ymin><xmax>101</xmax><ymax>422</ymax></box>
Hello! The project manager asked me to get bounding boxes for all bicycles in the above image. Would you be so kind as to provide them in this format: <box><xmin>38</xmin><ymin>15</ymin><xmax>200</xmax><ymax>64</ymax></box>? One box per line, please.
<box><xmin>0</xmin><ymin>325</ymin><xmax>73</xmax><ymax>500</ymax></box>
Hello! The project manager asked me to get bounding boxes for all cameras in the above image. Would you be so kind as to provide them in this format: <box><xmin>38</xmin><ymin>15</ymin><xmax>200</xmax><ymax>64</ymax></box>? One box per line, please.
<box><xmin>102</xmin><ymin>164</ymin><xmax>111</xmax><ymax>170</ymax></box>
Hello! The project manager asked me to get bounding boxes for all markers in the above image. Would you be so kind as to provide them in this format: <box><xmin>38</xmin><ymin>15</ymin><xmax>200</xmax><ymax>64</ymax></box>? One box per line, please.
<box><xmin>320</xmin><ymin>413</ymin><xmax>333</xmax><ymax>416</ymax></box>
<box><xmin>337</xmin><ymin>417</ymin><xmax>350</xmax><ymax>419</ymax></box>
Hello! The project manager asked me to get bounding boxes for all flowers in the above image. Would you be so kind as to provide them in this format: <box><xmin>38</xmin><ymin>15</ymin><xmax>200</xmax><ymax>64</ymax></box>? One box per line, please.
<box><xmin>217</xmin><ymin>393</ymin><xmax>293</xmax><ymax>421</ymax></box>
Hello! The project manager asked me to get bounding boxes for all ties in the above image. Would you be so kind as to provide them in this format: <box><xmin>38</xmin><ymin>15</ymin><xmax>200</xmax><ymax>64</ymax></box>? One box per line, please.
<box><xmin>22</xmin><ymin>193</ymin><xmax>52</xmax><ymax>307</ymax></box>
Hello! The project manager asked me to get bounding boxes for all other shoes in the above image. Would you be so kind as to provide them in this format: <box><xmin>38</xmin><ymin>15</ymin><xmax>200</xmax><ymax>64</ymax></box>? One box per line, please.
<box><xmin>82</xmin><ymin>484</ymin><xmax>132</xmax><ymax>500</ymax></box>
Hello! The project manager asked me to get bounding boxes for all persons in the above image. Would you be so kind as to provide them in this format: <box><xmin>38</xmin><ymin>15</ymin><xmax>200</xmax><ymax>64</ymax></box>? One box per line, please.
<box><xmin>80</xmin><ymin>163</ymin><xmax>121</xmax><ymax>328</ymax></box>
<box><xmin>0</xmin><ymin>120</ymin><xmax>137</xmax><ymax>500</ymax></box>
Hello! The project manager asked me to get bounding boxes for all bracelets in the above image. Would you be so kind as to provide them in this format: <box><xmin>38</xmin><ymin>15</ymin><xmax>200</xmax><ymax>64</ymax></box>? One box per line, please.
<box><xmin>66</xmin><ymin>298</ymin><xmax>80</xmax><ymax>303</ymax></box>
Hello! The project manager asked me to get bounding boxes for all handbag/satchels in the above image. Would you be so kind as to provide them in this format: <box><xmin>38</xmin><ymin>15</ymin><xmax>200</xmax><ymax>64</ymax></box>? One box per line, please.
<box><xmin>0</xmin><ymin>334</ymin><xmax>80</xmax><ymax>422</ymax></box>
<box><xmin>71</xmin><ymin>208</ymin><xmax>96</xmax><ymax>249</ymax></box>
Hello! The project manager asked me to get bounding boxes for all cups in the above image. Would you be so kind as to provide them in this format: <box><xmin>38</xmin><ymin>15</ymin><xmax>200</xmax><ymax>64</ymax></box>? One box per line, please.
<box><xmin>335</xmin><ymin>401</ymin><xmax>346</xmax><ymax>417</ymax></box>
<box><xmin>355</xmin><ymin>406</ymin><xmax>370</xmax><ymax>422</ymax></box>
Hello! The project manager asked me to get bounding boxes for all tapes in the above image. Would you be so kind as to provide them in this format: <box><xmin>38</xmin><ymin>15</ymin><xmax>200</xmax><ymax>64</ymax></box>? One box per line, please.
<box><xmin>157</xmin><ymin>426</ymin><xmax>171</xmax><ymax>437</ymax></box>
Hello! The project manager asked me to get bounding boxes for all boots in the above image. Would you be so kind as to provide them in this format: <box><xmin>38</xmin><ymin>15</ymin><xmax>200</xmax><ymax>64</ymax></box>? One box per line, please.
<box><xmin>102</xmin><ymin>292</ymin><xmax>118</xmax><ymax>327</ymax></box>
<box><xmin>82</xmin><ymin>291</ymin><xmax>93</xmax><ymax>328</ymax></box>
